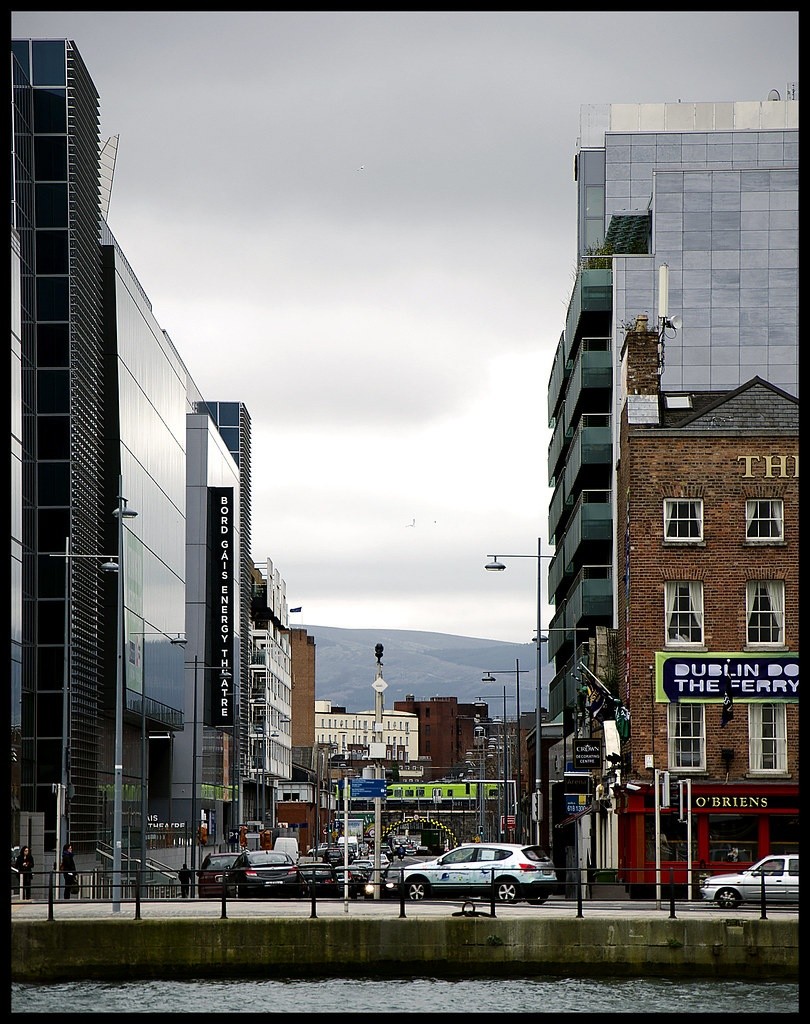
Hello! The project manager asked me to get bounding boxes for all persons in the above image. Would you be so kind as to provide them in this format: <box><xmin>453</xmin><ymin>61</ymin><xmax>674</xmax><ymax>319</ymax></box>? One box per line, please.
<box><xmin>15</xmin><ymin>846</ymin><xmax>34</xmax><ymax>900</ymax></box>
<box><xmin>179</xmin><ymin>864</ymin><xmax>192</xmax><ymax>898</ymax></box>
<box><xmin>62</xmin><ymin>843</ymin><xmax>76</xmax><ymax>899</ymax></box>
<box><xmin>398</xmin><ymin>844</ymin><xmax>404</xmax><ymax>859</ymax></box>
<box><xmin>768</xmin><ymin>861</ymin><xmax>786</xmax><ymax>876</ymax></box>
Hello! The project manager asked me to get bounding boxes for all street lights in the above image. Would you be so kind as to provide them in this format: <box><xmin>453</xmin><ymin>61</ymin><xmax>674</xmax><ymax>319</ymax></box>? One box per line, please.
<box><xmin>464</xmin><ymin>554</ymin><xmax>555</xmax><ymax>846</ymax></box>
<box><xmin>184</xmin><ymin>654</ymin><xmax>233</xmax><ymax>898</ymax></box>
<box><xmin>130</xmin><ymin>617</ymin><xmax>188</xmax><ymax>898</ymax></box>
<box><xmin>113</xmin><ymin>474</ymin><xmax>138</xmax><ymax>912</ymax></box>
<box><xmin>50</xmin><ymin>534</ymin><xmax>121</xmax><ymax>898</ymax></box>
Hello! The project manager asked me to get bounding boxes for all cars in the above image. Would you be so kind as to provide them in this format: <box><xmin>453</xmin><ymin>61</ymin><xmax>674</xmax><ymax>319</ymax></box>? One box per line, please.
<box><xmin>364</xmin><ymin>867</ymin><xmax>403</xmax><ymax>899</ymax></box>
<box><xmin>700</xmin><ymin>854</ymin><xmax>799</xmax><ymax>909</ymax></box>
<box><xmin>347</xmin><ymin>865</ymin><xmax>365</xmax><ymax>895</ymax></box>
<box><xmin>334</xmin><ymin>868</ymin><xmax>357</xmax><ymax>899</ymax></box>
<box><xmin>366</xmin><ymin>854</ymin><xmax>390</xmax><ymax>874</ymax></box>
<box><xmin>394</xmin><ymin>845</ymin><xmax>428</xmax><ymax>861</ymax></box>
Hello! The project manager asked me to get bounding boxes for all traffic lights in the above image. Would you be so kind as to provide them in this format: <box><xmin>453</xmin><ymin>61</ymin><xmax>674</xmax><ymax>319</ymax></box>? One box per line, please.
<box><xmin>664</xmin><ymin>773</ymin><xmax>683</xmax><ymax>821</ymax></box>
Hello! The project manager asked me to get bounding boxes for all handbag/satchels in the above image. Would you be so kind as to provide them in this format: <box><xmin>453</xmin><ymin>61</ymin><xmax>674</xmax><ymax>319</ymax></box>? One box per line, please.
<box><xmin>71</xmin><ymin>879</ymin><xmax>79</xmax><ymax>894</ymax></box>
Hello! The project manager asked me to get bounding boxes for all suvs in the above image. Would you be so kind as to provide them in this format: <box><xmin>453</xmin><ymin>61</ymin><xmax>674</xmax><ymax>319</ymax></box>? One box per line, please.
<box><xmin>337</xmin><ymin>845</ymin><xmax>355</xmax><ymax>864</ymax></box>
<box><xmin>223</xmin><ymin>851</ymin><xmax>300</xmax><ymax>898</ymax></box>
<box><xmin>399</xmin><ymin>843</ymin><xmax>558</xmax><ymax>905</ymax></box>
<box><xmin>322</xmin><ymin>848</ymin><xmax>344</xmax><ymax>867</ymax></box>
<box><xmin>198</xmin><ymin>853</ymin><xmax>244</xmax><ymax>897</ymax></box>
<box><xmin>351</xmin><ymin>860</ymin><xmax>374</xmax><ymax>881</ymax></box>
<box><xmin>381</xmin><ymin>846</ymin><xmax>393</xmax><ymax>862</ymax></box>
<box><xmin>298</xmin><ymin>862</ymin><xmax>339</xmax><ymax>898</ymax></box>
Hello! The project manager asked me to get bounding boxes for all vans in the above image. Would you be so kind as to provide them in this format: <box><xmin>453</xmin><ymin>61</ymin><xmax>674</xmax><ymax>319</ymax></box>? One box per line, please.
<box><xmin>337</xmin><ymin>837</ymin><xmax>369</xmax><ymax>859</ymax></box>
<box><xmin>307</xmin><ymin>843</ymin><xmax>337</xmax><ymax>857</ymax></box>
<box><xmin>274</xmin><ymin>838</ymin><xmax>298</xmax><ymax>864</ymax></box>
